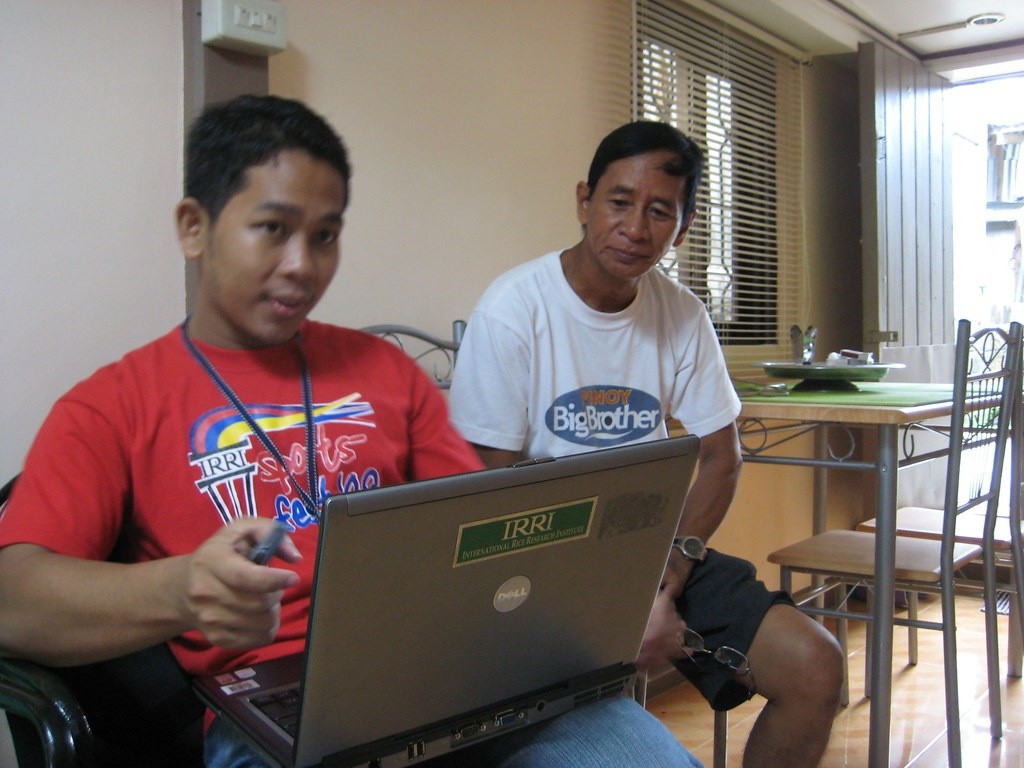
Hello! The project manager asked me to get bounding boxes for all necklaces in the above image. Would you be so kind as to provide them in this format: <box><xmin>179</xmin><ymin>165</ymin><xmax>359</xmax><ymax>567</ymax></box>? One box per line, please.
<box><xmin>179</xmin><ymin>316</ymin><xmax>322</xmax><ymax>527</ymax></box>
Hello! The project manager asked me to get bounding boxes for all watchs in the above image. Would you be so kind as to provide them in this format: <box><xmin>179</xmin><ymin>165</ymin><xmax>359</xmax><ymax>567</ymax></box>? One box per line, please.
<box><xmin>672</xmin><ymin>535</ymin><xmax>707</xmax><ymax>561</ymax></box>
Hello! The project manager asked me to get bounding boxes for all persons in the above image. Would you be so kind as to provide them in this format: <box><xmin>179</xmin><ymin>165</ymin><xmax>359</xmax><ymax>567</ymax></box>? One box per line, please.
<box><xmin>0</xmin><ymin>93</ymin><xmax>703</xmax><ymax>768</ymax></box>
<box><xmin>447</xmin><ymin>119</ymin><xmax>847</xmax><ymax>768</ymax></box>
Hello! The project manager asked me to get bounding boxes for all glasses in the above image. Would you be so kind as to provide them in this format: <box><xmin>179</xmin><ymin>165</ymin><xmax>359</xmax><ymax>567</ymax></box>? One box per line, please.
<box><xmin>673</xmin><ymin>628</ymin><xmax>751</xmax><ymax>701</ymax></box>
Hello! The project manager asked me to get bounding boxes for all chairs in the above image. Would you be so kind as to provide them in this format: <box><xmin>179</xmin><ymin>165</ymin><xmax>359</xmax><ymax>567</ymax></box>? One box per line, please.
<box><xmin>767</xmin><ymin>322</ymin><xmax>1022</xmax><ymax>768</ymax></box>
<box><xmin>356</xmin><ymin>320</ymin><xmax>728</xmax><ymax>768</ymax></box>
<box><xmin>855</xmin><ymin>324</ymin><xmax>1024</xmax><ymax>699</ymax></box>
<box><xmin>0</xmin><ymin>468</ymin><xmax>204</xmax><ymax>768</ymax></box>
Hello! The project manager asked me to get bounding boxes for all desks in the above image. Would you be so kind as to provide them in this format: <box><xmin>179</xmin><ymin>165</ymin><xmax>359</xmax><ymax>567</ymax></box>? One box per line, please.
<box><xmin>725</xmin><ymin>383</ymin><xmax>1001</xmax><ymax>706</ymax></box>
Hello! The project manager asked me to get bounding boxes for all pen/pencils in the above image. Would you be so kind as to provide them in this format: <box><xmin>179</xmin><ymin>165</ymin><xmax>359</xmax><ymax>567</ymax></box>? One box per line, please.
<box><xmin>246</xmin><ymin>523</ymin><xmax>291</xmax><ymax>563</ymax></box>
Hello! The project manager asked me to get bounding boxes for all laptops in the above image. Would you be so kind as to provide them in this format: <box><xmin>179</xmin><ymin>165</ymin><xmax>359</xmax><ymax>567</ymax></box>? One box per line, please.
<box><xmin>193</xmin><ymin>435</ymin><xmax>703</xmax><ymax>768</ymax></box>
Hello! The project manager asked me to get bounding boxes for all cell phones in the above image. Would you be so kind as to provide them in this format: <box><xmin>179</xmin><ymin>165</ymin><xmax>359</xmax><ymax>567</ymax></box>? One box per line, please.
<box><xmin>251</xmin><ymin>527</ymin><xmax>283</xmax><ymax>565</ymax></box>
